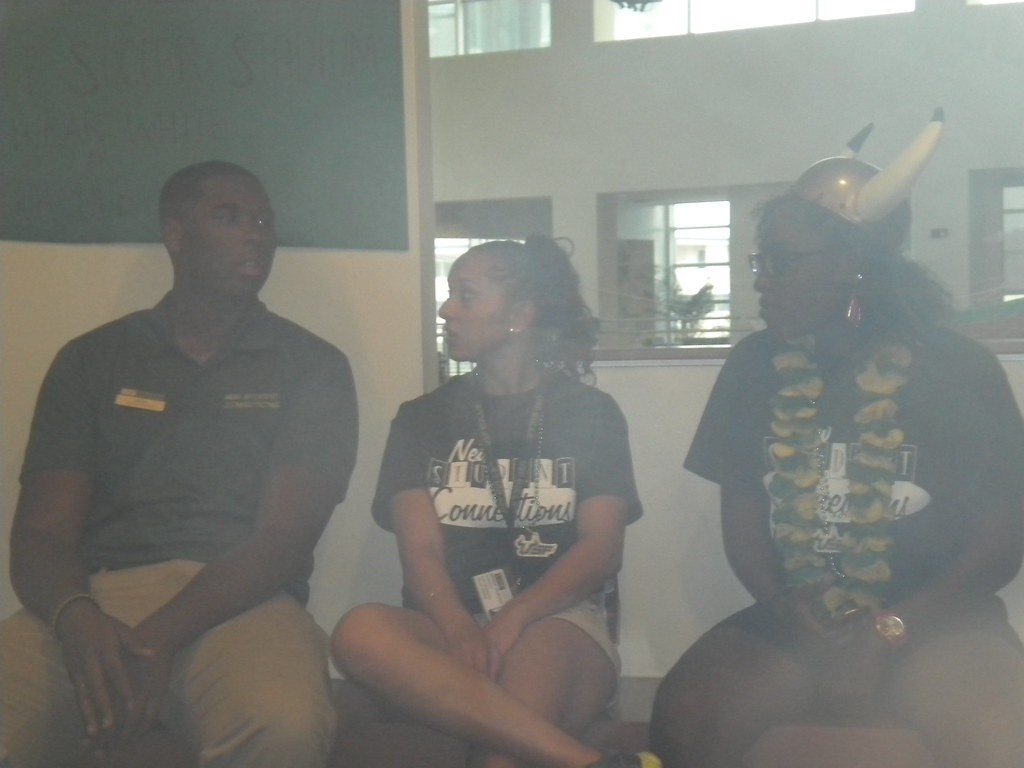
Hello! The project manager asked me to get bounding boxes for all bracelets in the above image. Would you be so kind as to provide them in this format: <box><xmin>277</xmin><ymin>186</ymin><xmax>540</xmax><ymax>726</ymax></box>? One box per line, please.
<box><xmin>51</xmin><ymin>593</ymin><xmax>102</xmax><ymax>643</ymax></box>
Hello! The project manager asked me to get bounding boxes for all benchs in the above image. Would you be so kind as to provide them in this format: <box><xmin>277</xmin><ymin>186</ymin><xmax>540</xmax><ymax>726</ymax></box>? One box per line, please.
<box><xmin>136</xmin><ymin>677</ymin><xmax>926</xmax><ymax>768</ymax></box>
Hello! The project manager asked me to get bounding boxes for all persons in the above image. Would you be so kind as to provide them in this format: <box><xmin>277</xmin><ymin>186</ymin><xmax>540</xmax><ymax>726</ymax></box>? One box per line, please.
<box><xmin>329</xmin><ymin>235</ymin><xmax>661</xmax><ymax>768</ymax></box>
<box><xmin>0</xmin><ymin>162</ymin><xmax>359</xmax><ymax>768</ymax></box>
<box><xmin>651</xmin><ymin>106</ymin><xmax>1023</xmax><ymax>768</ymax></box>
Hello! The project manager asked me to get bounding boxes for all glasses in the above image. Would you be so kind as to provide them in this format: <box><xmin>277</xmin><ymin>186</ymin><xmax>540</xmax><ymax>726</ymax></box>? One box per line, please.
<box><xmin>747</xmin><ymin>252</ymin><xmax>819</xmax><ymax>283</ymax></box>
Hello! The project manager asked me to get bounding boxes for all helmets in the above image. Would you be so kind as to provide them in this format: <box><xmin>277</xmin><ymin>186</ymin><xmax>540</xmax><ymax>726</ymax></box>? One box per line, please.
<box><xmin>790</xmin><ymin>107</ymin><xmax>945</xmax><ymax>249</ymax></box>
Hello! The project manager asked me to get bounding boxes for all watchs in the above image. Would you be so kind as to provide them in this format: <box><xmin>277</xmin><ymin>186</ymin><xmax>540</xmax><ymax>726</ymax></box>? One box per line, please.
<box><xmin>872</xmin><ymin>608</ymin><xmax>907</xmax><ymax>655</ymax></box>
